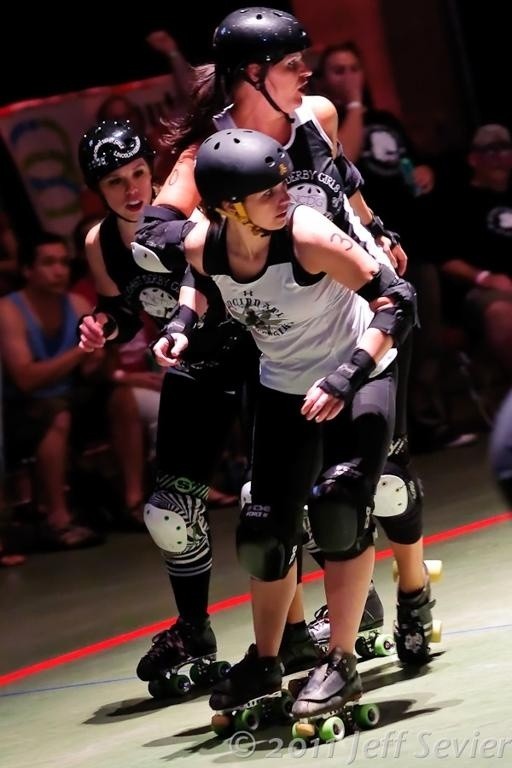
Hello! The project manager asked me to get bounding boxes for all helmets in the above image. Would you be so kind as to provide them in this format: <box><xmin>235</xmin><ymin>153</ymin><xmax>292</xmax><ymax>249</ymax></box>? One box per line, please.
<box><xmin>193</xmin><ymin>129</ymin><xmax>295</xmax><ymax>210</ymax></box>
<box><xmin>212</xmin><ymin>7</ymin><xmax>313</xmax><ymax>78</ymax></box>
<box><xmin>77</xmin><ymin>117</ymin><xmax>157</xmax><ymax>191</ymax></box>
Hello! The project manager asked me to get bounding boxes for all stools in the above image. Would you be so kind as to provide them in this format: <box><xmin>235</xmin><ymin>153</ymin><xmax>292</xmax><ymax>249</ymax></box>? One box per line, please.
<box><xmin>16</xmin><ymin>429</ymin><xmax>112</xmax><ymax>562</ymax></box>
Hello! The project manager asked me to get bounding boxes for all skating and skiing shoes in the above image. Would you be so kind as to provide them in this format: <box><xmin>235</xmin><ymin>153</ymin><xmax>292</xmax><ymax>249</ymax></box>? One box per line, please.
<box><xmin>137</xmin><ymin>614</ymin><xmax>232</xmax><ymax>703</ymax></box>
<box><xmin>392</xmin><ymin>563</ymin><xmax>444</xmax><ymax>678</ymax></box>
<box><xmin>208</xmin><ymin>580</ymin><xmax>398</xmax><ymax>746</ymax></box>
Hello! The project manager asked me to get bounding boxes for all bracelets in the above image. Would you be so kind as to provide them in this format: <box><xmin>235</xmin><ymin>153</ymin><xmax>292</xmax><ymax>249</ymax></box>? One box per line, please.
<box><xmin>164</xmin><ymin>49</ymin><xmax>179</xmax><ymax>61</ymax></box>
<box><xmin>346</xmin><ymin>345</ymin><xmax>376</xmax><ymax>378</ymax></box>
<box><xmin>476</xmin><ymin>270</ymin><xmax>490</xmax><ymax>286</ymax></box>
<box><xmin>171</xmin><ymin>301</ymin><xmax>200</xmax><ymax>328</ymax></box>
<box><xmin>362</xmin><ymin>215</ymin><xmax>387</xmax><ymax>238</ymax></box>
<box><xmin>345</xmin><ymin>98</ymin><xmax>363</xmax><ymax>110</ymax></box>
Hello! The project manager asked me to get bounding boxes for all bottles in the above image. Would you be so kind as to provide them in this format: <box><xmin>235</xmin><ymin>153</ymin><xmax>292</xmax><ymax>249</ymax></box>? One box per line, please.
<box><xmin>397</xmin><ymin>150</ymin><xmax>423</xmax><ymax>194</ymax></box>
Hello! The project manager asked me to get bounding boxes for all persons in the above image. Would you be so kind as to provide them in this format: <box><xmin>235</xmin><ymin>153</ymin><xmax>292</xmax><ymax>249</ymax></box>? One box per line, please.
<box><xmin>133</xmin><ymin>7</ymin><xmax>445</xmax><ymax>662</ymax></box>
<box><xmin>98</xmin><ymin>27</ymin><xmax>195</xmax><ymax>169</ymax></box>
<box><xmin>67</xmin><ymin>117</ymin><xmax>321</xmax><ymax>679</ymax></box>
<box><xmin>312</xmin><ymin>38</ymin><xmax>445</xmax><ymax>274</ymax></box>
<box><xmin>444</xmin><ymin>121</ymin><xmax>509</xmax><ymax>380</ymax></box>
<box><xmin>147</xmin><ymin>128</ymin><xmax>412</xmax><ymax>723</ymax></box>
<box><xmin>0</xmin><ymin>236</ymin><xmax>153</xmax><ymax>535</ymax></box>
<box><xmin>488</xmin><ymin>386</ymin><xmax>510</xmax><ymax>512</ymax></box>
<box><xmin>68</xmin><ymin>213</ymin><xmax>237</xmax><ymax>513</ymax></box>
